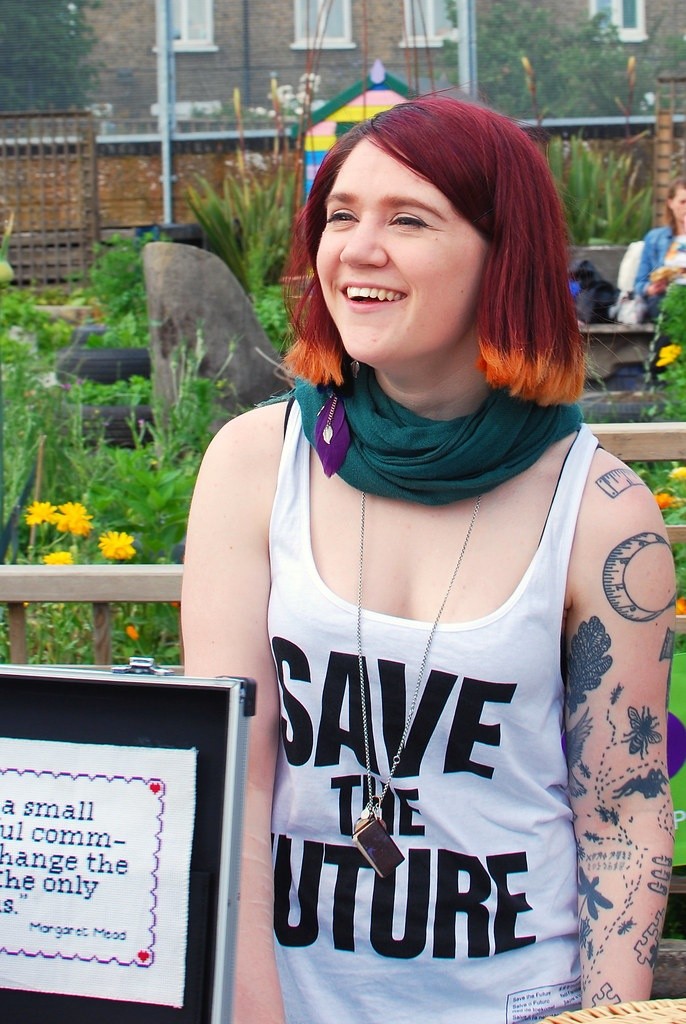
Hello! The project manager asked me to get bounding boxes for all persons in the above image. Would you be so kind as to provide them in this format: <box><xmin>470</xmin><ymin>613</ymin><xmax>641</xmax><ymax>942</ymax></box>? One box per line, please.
<box><xmin>182</xmin><ymin>95</ymin><xmax>676</xmax><ymax>1024</ymax></box>
<box><xmin>631</xmin><ymin>178</ymin><xmax>686</xmax><ymax>376</ymax></box>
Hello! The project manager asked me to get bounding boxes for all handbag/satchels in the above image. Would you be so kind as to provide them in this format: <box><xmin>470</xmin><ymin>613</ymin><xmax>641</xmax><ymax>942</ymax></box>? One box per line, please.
<box><xmin>617</xmin><ymin>241</ymin><xmax>648</xmax><ymax>326</ymax></box>
<box><xmin>568</xmin><ymin>259</ymin><xmax>619</xmax><ymax>325</ymax></box>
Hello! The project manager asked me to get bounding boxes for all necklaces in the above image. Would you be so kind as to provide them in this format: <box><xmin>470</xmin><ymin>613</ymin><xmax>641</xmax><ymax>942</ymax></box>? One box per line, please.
<box><xmin>352</xmin><ymin>488</ymin><xmax>483</xmax><ymax>878</ymax></box>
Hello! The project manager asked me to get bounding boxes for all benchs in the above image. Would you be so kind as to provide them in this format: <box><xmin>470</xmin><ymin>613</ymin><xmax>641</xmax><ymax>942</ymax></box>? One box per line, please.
<box><xmin>561</xmin><ymin>246</ymin><xmax>657</xmax><ymax>381</ymax></box>
